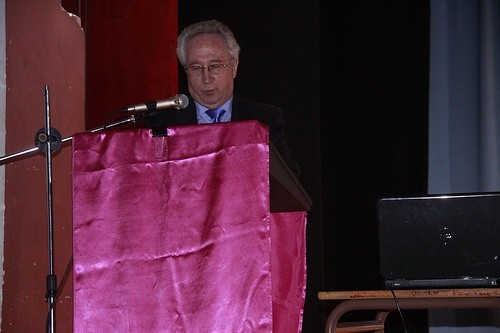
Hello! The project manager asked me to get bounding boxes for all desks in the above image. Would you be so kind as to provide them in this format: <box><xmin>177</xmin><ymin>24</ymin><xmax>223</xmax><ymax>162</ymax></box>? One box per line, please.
<box><xmin>317</xmin><ymin>288</ymin><xmax>500</xmax><ymax>333</ymax></box>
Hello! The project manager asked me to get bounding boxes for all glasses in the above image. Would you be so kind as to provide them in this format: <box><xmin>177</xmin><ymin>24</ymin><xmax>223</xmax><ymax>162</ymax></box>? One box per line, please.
<box><xmin>183</xmin><ymin>57</ymin><xmax>233</xmax><ymax>75</ymax></box>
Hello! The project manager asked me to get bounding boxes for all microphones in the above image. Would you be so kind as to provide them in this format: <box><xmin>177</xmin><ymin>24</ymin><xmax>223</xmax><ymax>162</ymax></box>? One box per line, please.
<box><xmin>120</xmin><ymin>94</ymin><xmax>188</xmax><ymax>113</ymax></box>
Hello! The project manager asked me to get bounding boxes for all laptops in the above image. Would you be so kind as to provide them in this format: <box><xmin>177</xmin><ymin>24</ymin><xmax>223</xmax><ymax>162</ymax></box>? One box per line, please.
<box><xmin>378</xmin><ymin>192</ymin><xmax>500</xmax><ymax>287</ymax></box>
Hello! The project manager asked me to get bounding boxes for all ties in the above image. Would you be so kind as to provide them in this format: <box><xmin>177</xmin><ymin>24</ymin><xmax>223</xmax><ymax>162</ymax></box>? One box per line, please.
<box><xmin>204</xmin><ymin>107</ymin><xmax>226</xmax><ymax>123</ymax></box>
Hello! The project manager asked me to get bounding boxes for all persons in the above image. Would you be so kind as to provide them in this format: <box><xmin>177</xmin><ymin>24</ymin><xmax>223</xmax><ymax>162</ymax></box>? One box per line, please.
<box><xmin>141</xmin><ymin>20</ymin><xmax>300</xmax><ymax>180</ymax></box>
